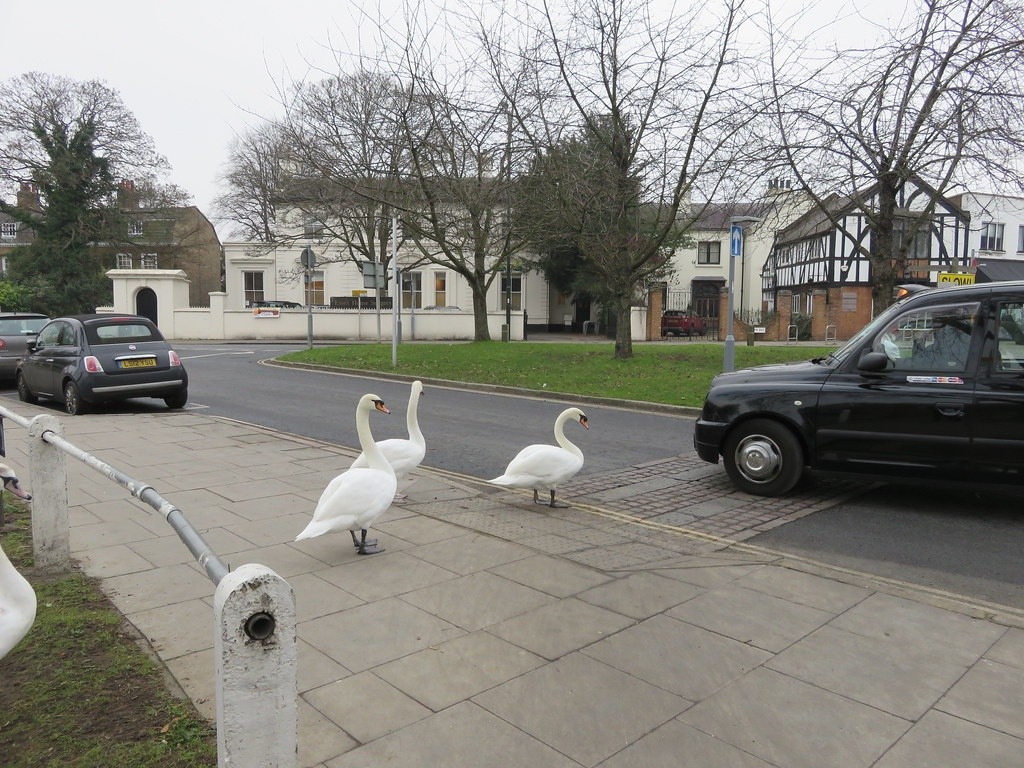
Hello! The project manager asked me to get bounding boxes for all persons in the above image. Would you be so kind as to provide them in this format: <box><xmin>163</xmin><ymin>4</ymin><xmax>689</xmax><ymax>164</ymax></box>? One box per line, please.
<box><xmin>904</xmin><ymin>309</ymin><xmax>968</xmax><ymax>371</ymax></box>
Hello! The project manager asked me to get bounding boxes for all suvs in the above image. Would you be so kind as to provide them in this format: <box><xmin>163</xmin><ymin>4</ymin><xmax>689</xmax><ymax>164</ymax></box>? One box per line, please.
<box><xmin>662</xmin><ymin>310</ymin><xmax>707</xmax><ymax>337</ymax></box>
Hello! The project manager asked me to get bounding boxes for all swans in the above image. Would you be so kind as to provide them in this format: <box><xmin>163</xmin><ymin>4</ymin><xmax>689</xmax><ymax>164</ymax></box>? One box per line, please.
<box><xmin>479</xmin><ymin>407</ymin><xmax>590</xmax><ymax>510</ymax></box>
<box><xmin>293</xmin><ymin>379</ymin><xmax>428</xmax><ymax>557</ymax></box>
<box><xmin>0</xmin><ymin>463</ymin><xmax>38</xmax><ymax>655</ymax></box>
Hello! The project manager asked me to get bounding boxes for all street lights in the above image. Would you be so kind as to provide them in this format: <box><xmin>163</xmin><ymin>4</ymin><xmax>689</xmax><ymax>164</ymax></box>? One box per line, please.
<box><xmin>300</xmin><ymin>245</ymin><xmax>313</xmax><ymax>349</ymax></box>
<box><xmin>723</xmin><ymin>215</ymin><xmax>761</xmax><ymax>377</ymax></box>
<box><xmin>499</xmin><ymin>100</ymin><xmax>511</xmax><ymax>340</ymax></box>
<box><xmin>969</xmin><ymin>249</ymin><xmax>991</xmax><ymax>275</ymax></box>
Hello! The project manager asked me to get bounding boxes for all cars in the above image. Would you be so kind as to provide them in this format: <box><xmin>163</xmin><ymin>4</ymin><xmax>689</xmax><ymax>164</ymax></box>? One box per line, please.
<box><xmin>692</xmin><ymin>278</ymin><xmax>1024</xmax><ymax>498</ymax></box>
<box><xmin>0</xmin><ymin>311</ymin><xmax>53</xmax><ymax>386</ymax></box>
<box><xmin>15</xmin><ymin>314</ymin><xmax>188</xmax><ymax>415</ymax></box>
